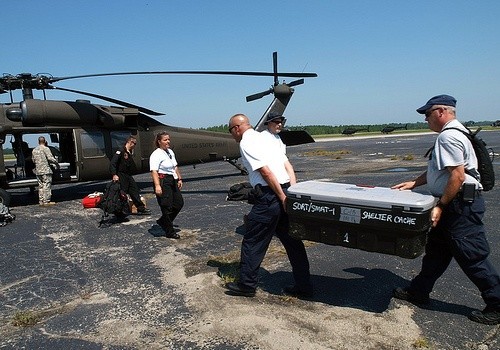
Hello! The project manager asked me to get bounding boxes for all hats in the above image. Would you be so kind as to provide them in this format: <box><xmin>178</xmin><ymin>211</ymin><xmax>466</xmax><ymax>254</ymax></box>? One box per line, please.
<box><xmin>264</xmin><ymin>112</ymin><xmax>285</xmax><ymax>126</ymax></box>
<box><xmin>416</xmin><ymin>95</ymin><xmax>457</xmax><ymax>114</ymax></box>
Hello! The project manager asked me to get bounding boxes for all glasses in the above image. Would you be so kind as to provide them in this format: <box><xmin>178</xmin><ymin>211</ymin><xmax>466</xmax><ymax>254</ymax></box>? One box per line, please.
<box><xmin>425</xmin><ymin>107</ymin><xmax>447</xmax><ymax>117</ymax></box>
<box><xmin>132</xmin><ymin>141</ymin><xmax>137</xmax><ymax>146</ymax></box>
<box><xmin>271</xmin><ymin>120</ymin><xmax>283</xmax><ymax>125</ymax></box>
<box><xmin>228</xmin><ymin>125</ymin><xmax>241</xmax><ymax>133</ymax></box>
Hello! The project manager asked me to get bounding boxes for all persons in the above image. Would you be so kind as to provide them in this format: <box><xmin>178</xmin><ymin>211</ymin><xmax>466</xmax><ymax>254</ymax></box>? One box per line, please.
<box><xmin>109</xmin><ymin>135</ymin><xmax>150</xmax><ymax>215</ymax></box>
<box><xmin>226</xmin><ymin>114</ymin><xmax>314</xmax><ymax>296</ymax></box>
<box><xmin>236</xmin><ymin>112</ymin><xmax>286</xmax><ymax>235</ymax></box>
<box><xmin>32</xmin><ymin>136</ymin><xmax>60</xmax><ymax>207</ymax></box>
<box><xmin>149</xmin><ymin>132</ymin><xmax>184</xmax><ymax>238</ymax></box>
<box><xmin>389</xmin><ymin>95</ymin><xmax>500</xmax><ymax>326</ymax></box>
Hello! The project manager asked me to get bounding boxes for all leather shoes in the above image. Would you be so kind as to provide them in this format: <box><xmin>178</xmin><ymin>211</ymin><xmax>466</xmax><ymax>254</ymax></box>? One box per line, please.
<box><xmin>137</xmin><ymin>208</ymin><xmax>151</xmax><ymax>212</ymax></box>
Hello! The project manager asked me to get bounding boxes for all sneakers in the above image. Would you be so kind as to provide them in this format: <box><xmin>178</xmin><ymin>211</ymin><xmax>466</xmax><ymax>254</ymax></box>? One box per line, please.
<box><xmin>285</xmin><ymin>283</ymin><xmax>313</xmax><ymax>297</ymax></box>
<box><xmin>226</xmin><ymin>279</ymin><xmax>256</xmax><ymax>297</ymax></box>
<box><xmin>47</xmin><ymin>202</ymin><xmax>56</xmax><ymax>205</ymax></box>
<box><xmin>469</xmin><ymin>304</ymin><xmax>499</xmax><ymax>325</ymax></box>
<box><xmin>394</xmin><ymin>285</ymin><xmax>431</xmax><ymax>309</ymax></box>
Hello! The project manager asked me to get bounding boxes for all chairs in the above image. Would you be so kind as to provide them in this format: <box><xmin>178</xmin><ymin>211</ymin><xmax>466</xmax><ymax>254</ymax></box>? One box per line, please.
<box><xmin>18</xmin><ymin>141</ymin><xmax>71</xmax><ymax>179</ymax></box>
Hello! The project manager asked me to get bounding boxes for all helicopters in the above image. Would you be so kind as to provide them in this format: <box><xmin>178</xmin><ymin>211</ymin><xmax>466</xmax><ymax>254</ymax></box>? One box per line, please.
<box><xmin>0</xmin><ymin>50</ymin><xmax>318</xmax><ymax>199</ymax></box>
<box><xmin>491</xmin><ymin>120</ymin><xmax>500</xmax><ymax>127</ymax></box>
<box><xmin>382</xmin><ymin>124</ymin><xmax>407</xmax><ymax>134</ymax></box>
<box><xmin>341</xmin><ymin>125</ymin><xmax>370</xmax><ymax>137</ymax></box>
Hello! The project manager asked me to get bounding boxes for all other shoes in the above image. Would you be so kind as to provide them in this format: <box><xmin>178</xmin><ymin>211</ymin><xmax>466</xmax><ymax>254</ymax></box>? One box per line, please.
<box><xmin>172</xmin><ymin>234</ymin><xmax>180</xmax><ymax>239</ymax></box>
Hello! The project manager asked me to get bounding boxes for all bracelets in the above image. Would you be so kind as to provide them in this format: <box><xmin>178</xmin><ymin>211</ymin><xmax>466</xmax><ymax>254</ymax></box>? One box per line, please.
<box><xmin>436</xmin><ymin>201</ymin><xmax>446</xmax><ymax>209</ymax></box>
<box><xmin>177</xmin><ymin>179</ymin><xmax>183</xmax><ymax>181</ymax></box>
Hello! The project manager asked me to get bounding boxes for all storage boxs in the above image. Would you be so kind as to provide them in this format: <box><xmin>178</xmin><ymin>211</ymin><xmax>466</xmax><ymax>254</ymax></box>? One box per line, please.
<box><xmin>284</xmin><ymin>179</ymin><xmax>438</xmax><ymax>259</ymax></box>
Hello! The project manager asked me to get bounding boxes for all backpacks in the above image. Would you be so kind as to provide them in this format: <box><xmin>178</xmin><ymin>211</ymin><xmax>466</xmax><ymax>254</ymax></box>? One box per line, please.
<box><xmin>437</xmin><ymin>124</ymin><xmax>495</xmax><ymax>192</ymax></box>
<box><xmin>227</xmin><ymin>182</ymin><xmax>253</xmax><ymax>201</ymax></box>
<box><xmin>98</xmin><ymin>182</ymin><xmax>124</xmax><ymax>217</ymax></box>
